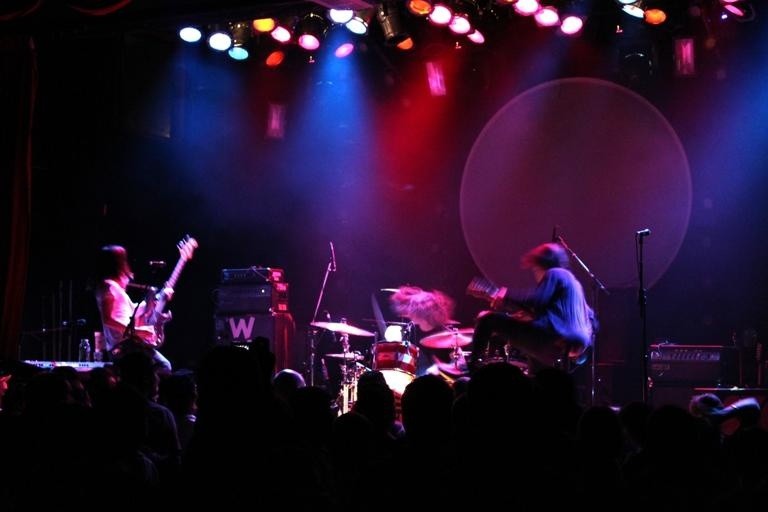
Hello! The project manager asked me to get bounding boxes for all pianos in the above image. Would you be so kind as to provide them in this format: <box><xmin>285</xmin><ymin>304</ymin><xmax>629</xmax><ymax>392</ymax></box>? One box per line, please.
<box><xmin>21</xmin><ymin>360</ymin><xmax>114</xmax><ymax>372</ymax></box>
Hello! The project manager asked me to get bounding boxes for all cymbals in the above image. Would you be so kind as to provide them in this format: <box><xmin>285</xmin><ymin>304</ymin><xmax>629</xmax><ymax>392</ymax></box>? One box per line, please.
<box><xmin>420</xmin><ymin>328</ymin><xmax>474</xmax><ymax>349</ymax></box>
<box><xmin>325</xmin><ymin>352</ymin><xmax>365</xmax><ymax>361</ymax></box>
<box><xmin>310</xmin><ymin>320</ymin><xmax>374</xmax><ymax>336</ymax></box>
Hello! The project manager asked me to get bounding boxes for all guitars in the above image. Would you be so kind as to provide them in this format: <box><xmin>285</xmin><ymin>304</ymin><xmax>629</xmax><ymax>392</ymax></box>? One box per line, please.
<box><xmin>127</xmin><ymin>234</ymin><xmax>198</xmax><ymax>347</ymax></box>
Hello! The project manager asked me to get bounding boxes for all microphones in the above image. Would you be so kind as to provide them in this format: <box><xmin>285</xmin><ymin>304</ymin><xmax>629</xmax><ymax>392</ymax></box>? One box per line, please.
<box><xmin>552</xmin><ymin>225</ymin><xmax>561</xmax><ymax>243</ymax></box>
<box><xmin>149</xmin><ymin>260</ymin><xmax>167</xmax><ymax>268</ymax></box>
<box><xmin>322</xmin><ymin>309</ymin><xmax>337</xmax><ymax>343</ymax></box>
<box><xmin>63</xmin><ymin>318</ymin><xmax>87</xmax><ymax>327</ymax></box>
<box><xmin>329</xmin><ymin>241</ymin><xmax>337</xmax><ymax>279</ymax></box>
<box><xmin>636</xmin><ymin>229</ymin><xmax>651</xmax><ymax>236</ymax></box>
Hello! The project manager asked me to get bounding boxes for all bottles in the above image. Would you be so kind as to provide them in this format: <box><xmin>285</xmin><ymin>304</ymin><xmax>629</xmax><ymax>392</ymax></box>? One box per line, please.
<box><xmin>79</xmin><ymin>338</ymin><xmax>91</xmax><ymax>361</ymax></box>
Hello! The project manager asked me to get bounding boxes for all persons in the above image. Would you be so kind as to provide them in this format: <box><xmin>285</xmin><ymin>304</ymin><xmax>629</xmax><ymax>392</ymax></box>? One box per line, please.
<box><xmin>403</xmin><ymin>290</ymin><xmax>464</xmax><ymax>377</ymax></box>
<box><xmin>468</xmin><ymin>242</ymin><xmax>598</xmax><ymax>378</ymax></box>
<box><xmin>0</xmin><ymin>346</ymin><xmax>766</xmax><ymax>511</ymax></box>
<box><xmin>362</xmin><ymin>284</ymin><xmax>424</xmax><ymax>360</ymax></box>
<box><xmin>95</xmin><ymin>246</ymin><xmax>173</xmax><ymax>371</ymax></box>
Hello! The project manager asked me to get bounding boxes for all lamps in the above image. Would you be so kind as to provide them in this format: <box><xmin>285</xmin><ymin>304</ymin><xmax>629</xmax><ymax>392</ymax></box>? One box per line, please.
<box><xmin>330</xmin><ymin>34</ymin><xmax>357</xmax><ymax>65</ymax></box>
<box><xmin>290</xmin><ymin>11</ymin><xmax>322</xmax><ymax>58</ymax></box>
<box><xmin>405</xmin><ymin>0</ymin><xmax>434</xmax><ymax>20</ymax></box>
<box><xmin>258</xmin><ymin>42</ymin><xmax>287</xmax><ymax>70</ymax></box>
<box><xmin>376</xmin><ymin>11</ymin><xmax>418</xmax><ymax>57</ymax></box>
<box><xmin>225</xmin><ymin>37</ymin><xmax>252</xmax><ymax>64</ymax></box>
<box><xmin>326</xmin><ymin>8</ymin><xmax>370</xmax><ymax>39</ymax></box>
<box><xmin>619</xmin><ymin>2</ymin><xmax>645</xmax><ymax>23</ymax></box>
<box><xmin>446</xmin><ymin>1</ymin><xmax>480</xmax><ymax>40</ymax></box>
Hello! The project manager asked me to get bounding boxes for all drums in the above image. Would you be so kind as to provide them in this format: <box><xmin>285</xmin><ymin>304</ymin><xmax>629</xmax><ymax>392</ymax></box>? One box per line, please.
<box><xmin>372</xmin><ymin>339</ymin><xmax>419</xmax><ymax>396</ymax></box>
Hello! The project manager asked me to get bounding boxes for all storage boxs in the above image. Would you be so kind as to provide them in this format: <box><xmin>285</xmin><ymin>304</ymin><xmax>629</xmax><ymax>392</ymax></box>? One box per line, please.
<box><xmin>215</xmin><ymin>281</ymin><xmax>290</xmax><ymax>312</ymax></box>
<box><xmin>213</xmin><ymin>315</ymin><xmax>297</xmax><ymax>372</ymax></box>
<box><xmin>218</xmin><ymin>265</ymin><xmax>286</xmax><ymax>283</ymax></box>
